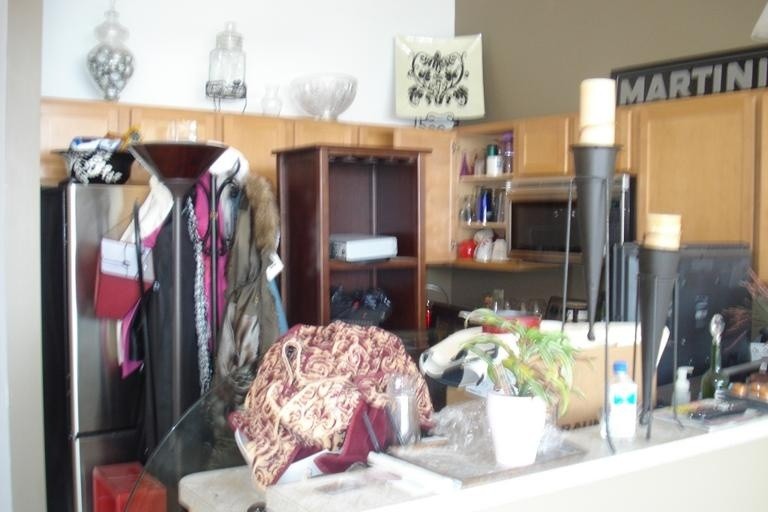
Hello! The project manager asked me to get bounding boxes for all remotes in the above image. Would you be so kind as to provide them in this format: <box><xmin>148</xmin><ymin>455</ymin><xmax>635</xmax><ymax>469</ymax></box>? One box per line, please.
<box><xmin>690</xmin><ymin>399</ymin><xmax>747</xmax><ymax>420</ymax></box>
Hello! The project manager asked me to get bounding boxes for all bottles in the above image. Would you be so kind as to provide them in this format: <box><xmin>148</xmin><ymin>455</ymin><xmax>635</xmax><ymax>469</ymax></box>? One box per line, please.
<box><xmin>385</xmin><ymin>372</ymin><xmax>420</xmax><ymax>445</ymax></box>
<box><xmin>700</xmin><ymin>338</ymin><xmax>728</xmax><ymax>399</ymax></box>
<box><xmin>485</xmin><ymin>139</ymin><xmax>513</xmax><ymax>177</ymax></box>
<box><xmin>609</xmin><ymin>363</ymin><xmax>638</xmax><ymax>444</ymax></box>
<box><xmin>458</xmin><ymin>186</ymin><xmax>505</xmax><ymax>222</ymax></box>
<box><xmin>259</xmin><ymin>84</ymin><xmax>283</xmax><ymax>116</ymax></box>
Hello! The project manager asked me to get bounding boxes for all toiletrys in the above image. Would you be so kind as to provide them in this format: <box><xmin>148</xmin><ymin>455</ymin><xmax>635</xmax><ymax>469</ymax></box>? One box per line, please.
<box><xmin>457</xmin><ymin>133</ymin><xmax>513</xmax><ymax>226</ymax></box>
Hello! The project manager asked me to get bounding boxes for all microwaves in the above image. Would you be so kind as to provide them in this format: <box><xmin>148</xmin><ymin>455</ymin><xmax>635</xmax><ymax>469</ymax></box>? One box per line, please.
<box><xmin>506</xmin><ymin>173</ymin><xmax>629</xmax><ymax>260</ymax></box>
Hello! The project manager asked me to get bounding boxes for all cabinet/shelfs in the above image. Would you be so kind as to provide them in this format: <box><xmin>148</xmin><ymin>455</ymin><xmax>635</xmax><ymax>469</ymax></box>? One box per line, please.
<box><xmin>458</xmin><ymin>116</ymin><xmax>521</xmax><ymax>265</ymax></box>
<box><xmin>269</xmin><ymin>142</ymin><xmax>436</xmax><ymax>360</ymax></box>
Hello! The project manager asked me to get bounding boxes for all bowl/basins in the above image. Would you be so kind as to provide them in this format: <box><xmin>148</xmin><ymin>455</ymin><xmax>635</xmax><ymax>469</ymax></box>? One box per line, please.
<box><xmin>124</xmin><ymin>142</ymin><xmax>230</xmax><ymax>179</ymax></box>
<box><xmin>47</xmin><ymin>145</ymin><xmax>137</xmax><ymax>184</ymax></box>
<box><xmin>289</xmin><ymin>72</ymin><xmax>360</xmax><ymax>122</ymax></box>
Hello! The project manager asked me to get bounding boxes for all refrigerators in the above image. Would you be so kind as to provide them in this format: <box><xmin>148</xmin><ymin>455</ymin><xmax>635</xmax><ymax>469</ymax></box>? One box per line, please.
<box><xmin>45</xmin><ymin>178</ymin><xmax>215</xmax><ymax>512</ymax></box>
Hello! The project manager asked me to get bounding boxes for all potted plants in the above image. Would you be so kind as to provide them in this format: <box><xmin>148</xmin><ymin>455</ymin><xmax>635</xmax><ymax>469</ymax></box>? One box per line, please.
<box><xmin>450</xmin><ymin>303</ymin><xmax>587</xmax><ymax>471</ymax></box>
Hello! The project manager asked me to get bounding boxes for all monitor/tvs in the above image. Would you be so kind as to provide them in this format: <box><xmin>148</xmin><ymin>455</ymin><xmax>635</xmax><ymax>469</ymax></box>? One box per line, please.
<box><xmin>611</xmin><ymin>243</ymin><xmax>752</xmax><ymax>388</ymax></box>
